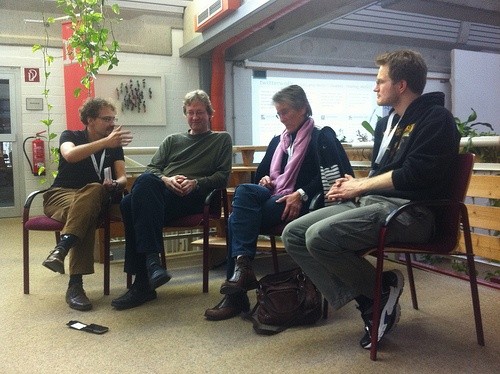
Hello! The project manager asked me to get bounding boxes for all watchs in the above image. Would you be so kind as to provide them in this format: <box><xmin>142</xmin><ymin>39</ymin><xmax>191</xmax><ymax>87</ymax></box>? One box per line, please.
<box><xmin>297</xmin><ymin>188</ymin><xmax>308</xmax><ymax>202</ymax></box>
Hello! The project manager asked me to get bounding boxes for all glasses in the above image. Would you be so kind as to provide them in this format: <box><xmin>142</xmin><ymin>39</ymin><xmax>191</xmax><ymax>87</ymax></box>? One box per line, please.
<box><xmin>276</xmin><ymin>104</ymin><xmax>299</xmax><ymax>119</ymax></box>
<box><xmin>96</xmin><ymin>115</ymin><xmax>116</xmax><ymax>123</ymax></box>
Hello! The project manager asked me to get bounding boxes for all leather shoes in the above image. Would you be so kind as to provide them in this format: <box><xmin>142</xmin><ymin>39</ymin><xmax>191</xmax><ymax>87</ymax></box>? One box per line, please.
<box><xmin>42</xmin><ymin>247</ymin><xmax>66</xmax><ymax>274</ymax></box>
<box><xmin>66</xmin><ymin>283</ymin><xmax>92</xmax><ymax>310</ymax></box>
<box><xmin>147</xmin><ymin>262</ymin><xmax>172</xmax><ymax>289</ymax></box>
<box><xmin>110</xmin><ymin>282</ymin><xmax>157</xmax><ymax>309</ymax></box>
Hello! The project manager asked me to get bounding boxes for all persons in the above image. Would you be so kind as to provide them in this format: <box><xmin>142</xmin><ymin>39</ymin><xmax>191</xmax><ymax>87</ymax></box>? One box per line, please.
<box><xmin>203</xmin><ymin>84</ymin><xmax>355</xmax><ymax>320</ymax></box>
<box><xmin>280</xmin><ymin>50</ymin><xmax>462</xmax><ymax>349</ymax></box>
<box><xmin>111</xmin><ymin>90</ymin><xmax>232</xmax><ymax>308</ymax></box>
<box><xmin>42</xmin><ymin>97</ymin><xmax>134</xmax><ymax>310</ymax></box>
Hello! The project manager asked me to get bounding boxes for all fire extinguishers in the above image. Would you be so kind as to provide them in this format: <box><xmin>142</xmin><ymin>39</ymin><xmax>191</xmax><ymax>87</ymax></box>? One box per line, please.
<box><xmin>32</xmin><ymin>130</ymin><xmax>47</xmax><ymax>176</ymax></box>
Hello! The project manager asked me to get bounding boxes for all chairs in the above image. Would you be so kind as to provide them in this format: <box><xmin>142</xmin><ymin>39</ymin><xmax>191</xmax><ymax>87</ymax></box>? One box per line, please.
<box><xmin>23</xmin><ymin>184</ymin><xmax>129</xmax><ymax>296</ymax></box>
<box><xmin>122</xmin><ymin>172</ymin><xmax>228</xmax><ymax>293</ymax></box>
<box><xmin>253</xmin><ymin>191</ymin><xmax>326</xmax><ymax>275</ymax></box>
<box><xmin>322</xmin><ymin>151</ymin><xmax>485</xmax><ymax>361</ymax></box>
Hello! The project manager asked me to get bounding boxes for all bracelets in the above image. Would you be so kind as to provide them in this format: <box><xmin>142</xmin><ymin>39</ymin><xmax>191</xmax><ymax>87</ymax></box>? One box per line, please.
<box><xmin>194</xmin><ymin>179</ymin><xmax>200</xmax><ymax>190</ymax></box>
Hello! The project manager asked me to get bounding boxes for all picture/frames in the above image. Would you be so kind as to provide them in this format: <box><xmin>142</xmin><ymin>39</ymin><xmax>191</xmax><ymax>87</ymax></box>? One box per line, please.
<box><xmin>92</xmin><ymin>71</ymin><xmax>167</xmax><ymax>126</ymax></box>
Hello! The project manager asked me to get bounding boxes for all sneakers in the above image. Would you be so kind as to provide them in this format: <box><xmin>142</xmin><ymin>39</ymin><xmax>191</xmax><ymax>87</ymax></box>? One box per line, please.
<box><xmin>380</xmin><ymin>268</ymin><xmax>405</xmax><ymax>334</ymax></box>
<box><xmin>359</xmin><ymin>284</ymin><xmax>399</xmax><ymax>349</ymax></box>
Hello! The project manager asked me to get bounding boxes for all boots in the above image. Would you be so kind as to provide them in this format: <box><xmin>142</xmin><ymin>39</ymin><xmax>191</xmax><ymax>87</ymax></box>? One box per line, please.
<box><xmin>204</xmin><ymin>293</ymin><xmax>250</xmax><ymax>320</ymax></box>
<box><xmin>220</xmin><ymin>257</ymin><xmax>258</xmax><ymax>295</ymax></box>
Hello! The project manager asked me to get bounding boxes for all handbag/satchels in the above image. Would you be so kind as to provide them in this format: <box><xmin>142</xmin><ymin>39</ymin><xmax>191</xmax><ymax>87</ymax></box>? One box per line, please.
<box><xmin>256</xmin><ymin>267</ymin><xmax>321</xmax><ymax>327</ymax></box>
